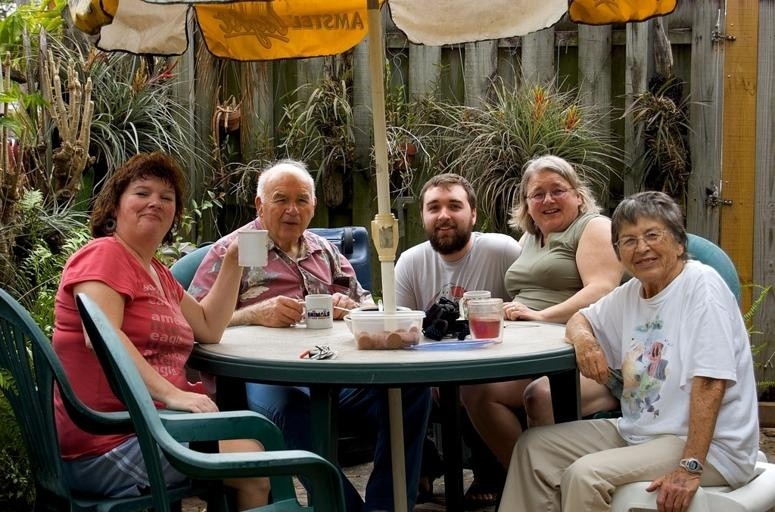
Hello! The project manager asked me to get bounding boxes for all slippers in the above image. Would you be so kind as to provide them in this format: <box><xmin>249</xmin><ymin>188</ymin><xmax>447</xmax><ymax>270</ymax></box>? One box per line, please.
<box><xmin>466</xmin><ymin>479</ymin><xmax>500</xmax><ymax>506</ymax></box>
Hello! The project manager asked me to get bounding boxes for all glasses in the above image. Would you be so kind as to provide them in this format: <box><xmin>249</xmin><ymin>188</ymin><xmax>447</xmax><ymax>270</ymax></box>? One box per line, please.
<box><xmin>614</xmin><ymin>230</ymin><xmax>673</xmax><ymax>250</ymax></box>
<box><xmin>523</xmin><ymin>187</ymin><xmax>575</xmax><ymax>202</ymax></box>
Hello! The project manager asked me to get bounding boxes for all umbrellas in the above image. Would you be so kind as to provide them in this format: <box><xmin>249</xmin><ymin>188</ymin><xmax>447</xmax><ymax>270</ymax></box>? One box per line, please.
<box><xmin>63</xmin><ymin>1</ymin><xmax>677</xmax><ymax>512</ymax></box>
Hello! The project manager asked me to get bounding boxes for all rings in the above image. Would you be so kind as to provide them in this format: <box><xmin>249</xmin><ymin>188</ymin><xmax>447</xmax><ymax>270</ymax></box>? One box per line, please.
<box><xmin>512</xmin><ymin>306</ymin><xmax>516</xmax><ymax>311</ymax></box>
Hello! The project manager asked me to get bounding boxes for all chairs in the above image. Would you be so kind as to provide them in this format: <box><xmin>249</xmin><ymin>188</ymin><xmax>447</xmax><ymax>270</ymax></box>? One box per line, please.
<box><xmin>1</xmin><ymin>286</ymin><xmax>297</xmax><ymax>512</ymax></box>
<box><xmin>169</xmin><ymin>240</ymin><xmax>364</xmax><ymax>443</ymax></box>
<box><xmin>76</xmin><ymin>291</ymin><xmax>372</xmax><ymax>511</ymax></box>
<box><xmin>684</xmin><ymin>233</ymin><xmax>741</xmax><ymax>296</ymax></box>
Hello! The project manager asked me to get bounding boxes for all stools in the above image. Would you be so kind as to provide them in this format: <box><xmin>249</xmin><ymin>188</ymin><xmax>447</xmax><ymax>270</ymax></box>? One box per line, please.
<box><xmin>611</xmin><ymin>461</ymin><xmax>775</xmax><ymax>510</ymax></box>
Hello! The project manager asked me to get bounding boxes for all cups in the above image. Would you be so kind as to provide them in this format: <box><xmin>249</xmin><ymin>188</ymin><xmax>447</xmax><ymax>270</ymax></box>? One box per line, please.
<box><xmin>237</xmin><ymin>228</ymin><xmax>268</xmax><ymax>268</ymax></box>
<box><xmin>469</xmin><ymin>299</ymin><xmax>505</xmax><ymax>341</ymax></box>
<box><xmin>459</xmin><ymin>290</ymin><xmax>491</xmax><ymax>321</ymax></box>
<box><xmin>296</xmin><ymin>294</ymin><xmax>333</xmax><ymax>330</ymax></box>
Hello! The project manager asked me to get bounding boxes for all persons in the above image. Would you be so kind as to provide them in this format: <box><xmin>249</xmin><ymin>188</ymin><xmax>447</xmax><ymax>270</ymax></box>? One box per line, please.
<box><xmin>457</xmin><ymin>154</ymin><xmax>623</xmax><ymax>473</ymax></box>
<box><xmin>48</xmin><ymin>150</ymin><xmax>272</xmax><ymax>511</ymax></box>
<box><xmin>495</xmin><ymin>190</ymin><xmax>763</xmax><ymax>512</ymax></box>
<box><xmin>388</xmin><ymin>172</ymin><xmax>522</xmax><ymax>508</ymax></box>
<box><xmin>186</xmin><ymin>159</ymin><xmax>434</xmax><ymax>512</ymax></box>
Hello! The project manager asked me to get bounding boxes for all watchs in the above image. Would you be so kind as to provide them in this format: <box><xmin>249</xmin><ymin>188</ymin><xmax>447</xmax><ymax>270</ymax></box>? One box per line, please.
<box><xmin>679</xmin><ymin>456</ymin><xmax>705</xmax><ymax>478</ymax></box>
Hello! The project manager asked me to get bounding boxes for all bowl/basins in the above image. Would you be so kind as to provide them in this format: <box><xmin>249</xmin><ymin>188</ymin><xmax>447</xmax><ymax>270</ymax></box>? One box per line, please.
<box><xmin>343</xmin><ymin>305</ymin><xmax>413</xmax><ymax>336</ymax></box>
